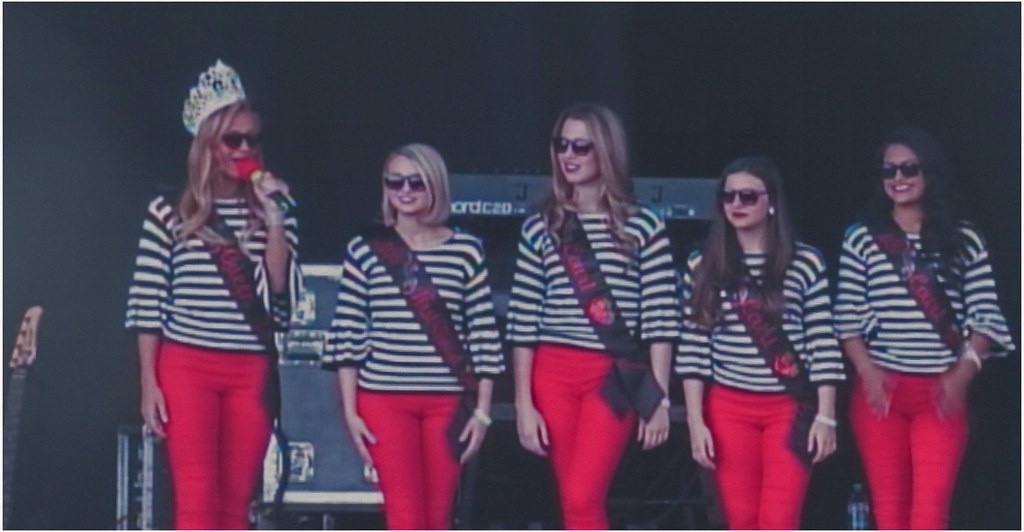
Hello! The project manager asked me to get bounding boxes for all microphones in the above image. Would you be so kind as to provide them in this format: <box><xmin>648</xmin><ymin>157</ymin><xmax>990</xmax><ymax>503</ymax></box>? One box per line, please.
<box><xmin>238</xmin><ymin>158</ymin><xmax>296</xmax><ymax>212</ymax></box>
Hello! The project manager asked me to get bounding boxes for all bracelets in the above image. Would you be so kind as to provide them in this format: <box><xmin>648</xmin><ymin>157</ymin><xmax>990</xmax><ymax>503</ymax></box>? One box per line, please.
<box><xmin>263</xmin><ymin>210</ymin><xmax>283</xmax><ymax>225</ymax></box>
<box><xmin>473</xmin><ymin>406</ymin><xmax>492</xmax><ymax>426</ymax></box>
<box><xmin>660</xmin><ymin>396</ymin><xmax>670</xmax><ymax>408</ymax></box>
<box><xmin>961</xmin><ymin>347</ymin><xmax>983</xmax><ymax>371</ymax></box>
<box><xmin>814</xmin><ymin>414</ymin><xmax>837</xmax><ymax>427</ymax></box>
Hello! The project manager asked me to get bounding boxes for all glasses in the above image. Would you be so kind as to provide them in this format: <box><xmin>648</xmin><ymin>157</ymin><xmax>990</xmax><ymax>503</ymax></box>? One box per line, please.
<box><xmin>554</xmin><ymin>137</ymin><xmax>595</xmax><ymax>156</ymax></box>
<box><xmin>722</xmin><ymin>189</ymin><xmax>769</xmax><ymax>206</ymax></box>
<box><xmin>221</xmin><ymin>130</ymin><xmax>264</xmax><ymax>150</ymax></box>
<box><xmin>881</xmin><ymin>163</ymin><xmax>921</xmax><ymax>178</ymax></box>
<box><xmin>383</xmin><ymin>172</ymin><xmax>426</xmax><ymax>192</ymax></box>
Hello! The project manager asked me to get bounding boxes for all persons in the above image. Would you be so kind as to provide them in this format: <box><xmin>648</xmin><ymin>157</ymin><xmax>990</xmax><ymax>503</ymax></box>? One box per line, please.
<box><xmin>319</xmin><ymin>143</ymin><xmax>506</xmax><ymax>530</ymax></box>
<box><xmin>508</xmin><ymin>99</ymin><xmax>682</xmax><ymax>530</ymax></box>
<box><xmin>676</xmin><ymin>151</ymin><xmax>848</xmax><ymax>529</ymax></box>
<box><xmin>836</xmin><ymin>126</ymin><xmax>1017</xmax><ymax>531</ymax></box>
<box><xmin>121</xmin><ymin>55</ymin><xmax>303</xmax><ymax>530</ymax></box>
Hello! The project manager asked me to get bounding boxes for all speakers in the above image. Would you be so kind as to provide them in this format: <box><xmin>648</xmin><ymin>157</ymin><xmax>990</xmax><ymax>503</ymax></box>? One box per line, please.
<box><xmin>272</xmin><ymin>357</ymin><xmax>385</xmax><ymax>507</ymax></box>
